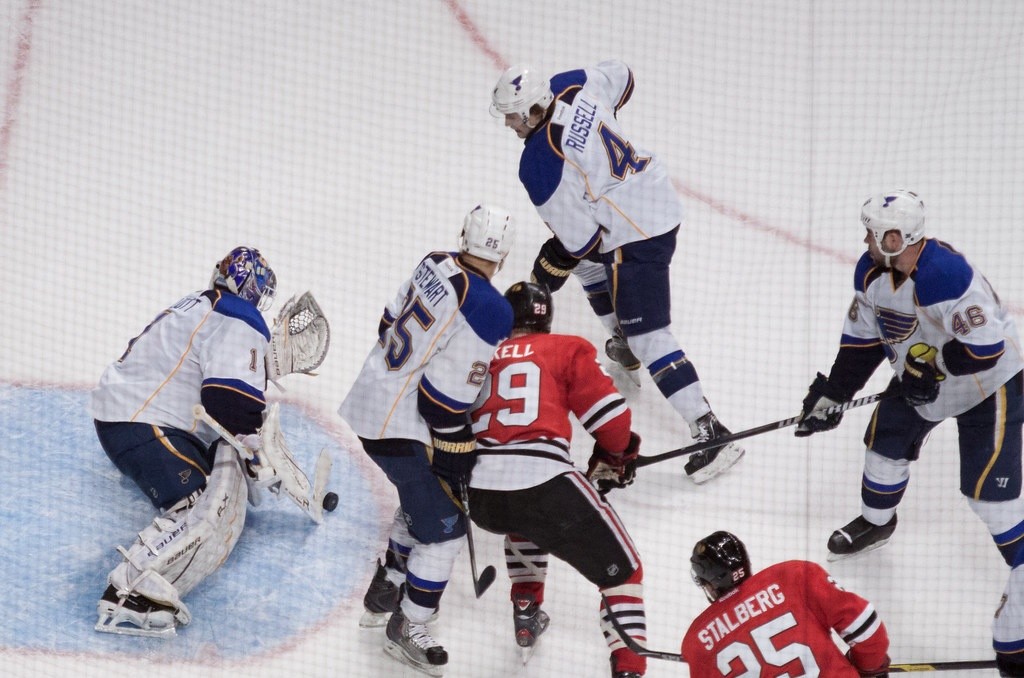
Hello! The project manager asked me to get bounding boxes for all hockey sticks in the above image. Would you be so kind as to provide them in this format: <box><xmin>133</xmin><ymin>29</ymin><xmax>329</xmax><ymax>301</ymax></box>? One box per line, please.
<box><xmin>635</xmin><ymin>384</ymin><xmax>904</xmax><ymax>468</ymax></box>
<box><xmin>460</xmin><ymin>480</ymin><xmax>497</xmax><ymax>600</ymax></box>
<box><xmin>600</xmin><ymin>593</ymin><xmax>1002</xmax><ymax>672</ymax></box>
<box><xmin>192</xmin><ymin>404</ymin><xmax>333</xmax><ymax>525</ymax></box>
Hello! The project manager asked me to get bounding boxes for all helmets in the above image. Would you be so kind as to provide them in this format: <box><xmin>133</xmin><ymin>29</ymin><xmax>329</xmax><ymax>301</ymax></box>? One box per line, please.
<box><xmin>690</xmin><ymin>531</ymin><xmax>749</xmax><ymax>604</ymax></box>
<box><xmin>860</xmin><ymin>189</ymin><xmax>927</xmax><ymax>256</ymax></box>
<box><xmin>459</xmin><ymin>201</ymin><xmax>515</xmax><ymax>263</ymax></box>
<box><xmin>488</xmin><ymin>63</ymin><xmax>554</xmax><ymax>128</ymax></box>
<box><xmin>219</xmin><ymin>246</ymin><xmax>279</xmax><ymax>315</ymax></box>
<box><xmin>504</xmin><ymin>281</ymin><xmax>553</xmax><ymax>334</ymax></box>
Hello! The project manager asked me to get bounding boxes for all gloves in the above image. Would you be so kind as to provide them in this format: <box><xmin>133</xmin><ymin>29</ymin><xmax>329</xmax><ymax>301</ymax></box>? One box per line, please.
<box><xmin>793</xmin><ymin>371</ymin><xmax>848</xmax><ymax>438</ymax></box>
<box><xmin>237</xmin><ymin>433</ymin><xmax>262</xmax><ymax>478</ymax></box>
<box><xmin>432</xmin><ymin>425</ymin><xmax>478</xmax><ymax>481</ymax></box>
<box><xmin>586</xmin><ymin>431</ymin><xmax>641</xmax><ymax>495</ymax></box>
<box><xmin>531</xmin><ymin>238</ymin><xmax>580</xmax><ymax>294</ymax></box>
<box><xmin>901</xmin><ymin>353</ymin><xmax>940</xmax><ymax>406</ymax></box>
<box><xmin>844</xmin><ymin>650</ymin><xmax>891</xmax><ymax>678</ymax></box>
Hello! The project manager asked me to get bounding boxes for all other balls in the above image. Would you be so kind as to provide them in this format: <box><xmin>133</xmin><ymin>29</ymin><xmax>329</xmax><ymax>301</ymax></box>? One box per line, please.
<box><xmin>323</xmin><ymin>492</ymin><xmax>339</xmax><ymax>512</ymax></box>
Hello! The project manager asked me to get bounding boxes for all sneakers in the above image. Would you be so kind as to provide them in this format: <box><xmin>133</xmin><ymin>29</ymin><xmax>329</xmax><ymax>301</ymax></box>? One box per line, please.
<box><xmin>827</xmin><ymin>513</ymin><xmax>898</xmax><ymax>562</ymax></box>
<box><xmin>684</xmin><ymin>396</ymin><xmax>745</xmax><ymax>485</ymax></box>
<box><xmin>513</xmin><ymin>594</ymin><xmax>550</xmax><ymax>665</ymax></box>
<box><xmin>94</xmin><ymin>583</ymin><xmax>177</xmax><ymax>640</ymax></box>
<box><xmin>605</xmin><ymin>325</ymin><xmax>642</xmax><ymax>387</ymax></box>
<box><xmin>358</xmin><ymin>559</ymin><xmax>449</xmax><ymax>678</ymax></box>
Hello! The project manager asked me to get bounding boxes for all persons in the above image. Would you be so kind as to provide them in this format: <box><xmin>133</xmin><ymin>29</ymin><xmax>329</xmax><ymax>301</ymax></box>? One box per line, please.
<box><xmin>796</xmin><ymin>188</ymin><xmax>1024</xmax><ymax>678</ymax></box>
<box><xmin>92</xmin><ymin>245</ymin><xmax>329</xmax><ymax>641</ymax></box>
<box><xmin>337</xmin><ymin>202</ymin><xmax>515</xmax><ymax>677</ymax></box>
<box><xmin>465</xmin><ymin>282</ymin><xmax>648</xmax><ymax>678</ymax></box>
<box><xmin>681</xmin><ymin>532</ymin><xmax>890</xmax><ymax>678</ymax></box>
<box><xmin>489</xmin><ymin>59</ymin><xmax>745</xmax><ymax>482</ymax></box>
